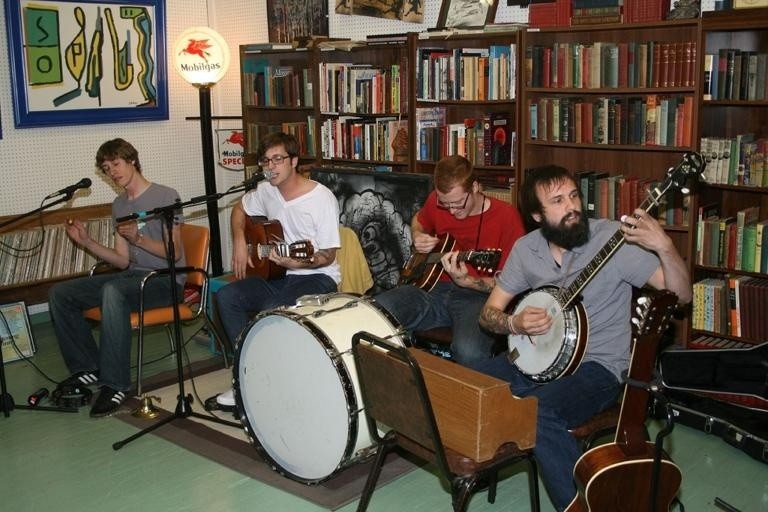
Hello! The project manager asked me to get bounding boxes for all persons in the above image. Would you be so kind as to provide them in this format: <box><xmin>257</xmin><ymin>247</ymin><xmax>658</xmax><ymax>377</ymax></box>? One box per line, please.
<box><xmin>216</xmin><ymin>132</ymin><xmax>341</xmax><ymax>405</ymax></box>
<box><xmin>476</xmin><ymin>164</ymin><xmax>692</xmax><ymax>510</ymax></box>
<box><xmin>373</xmin><ymin>155</ymin><xmax>526</xmax><ymax>369</ymax></box>
<box><xmin>48</xmin><ymin>135</ymin><xmax>188</xmax><ymax>419</ymax></box>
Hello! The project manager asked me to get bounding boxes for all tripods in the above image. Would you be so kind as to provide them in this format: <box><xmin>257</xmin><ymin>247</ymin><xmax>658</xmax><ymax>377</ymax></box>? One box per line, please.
<box><xmin>113</xmin><ymin>217</ymin><xmax>247</xmax><ymax>450</ymax></box>
<box><xmin>0</xmin><ymin>336</ymin><xmax>80</xmax><ymax>417</ymax></box>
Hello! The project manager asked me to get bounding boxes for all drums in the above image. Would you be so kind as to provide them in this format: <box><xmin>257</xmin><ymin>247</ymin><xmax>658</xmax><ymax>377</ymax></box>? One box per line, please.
<box><xmin>232</xmin><ymin>292</ymin><xmax>412</xmax><ymax>485</ymax></box>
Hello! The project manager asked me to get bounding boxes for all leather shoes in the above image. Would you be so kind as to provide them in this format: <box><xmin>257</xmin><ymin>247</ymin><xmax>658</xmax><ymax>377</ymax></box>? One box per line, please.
<box><xmin>217</xmin><ymin>387</ymin><xmax>237</xmax><ymax>406</ymax></box>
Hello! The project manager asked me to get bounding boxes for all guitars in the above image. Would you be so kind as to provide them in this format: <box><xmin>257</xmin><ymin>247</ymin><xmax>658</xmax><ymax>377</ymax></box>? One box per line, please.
<box><xmin>243</xmin><ymin>216</ymin><xmax>314</xmax><ymax>280</ymax></box>
<box><xmin>563</xmin><ymin>289</ymin><xmax>680</xmax><ymax>512</ymax></box>
<box><xmin>397</xmin><ymin>233</ymin><xmax>502</xmax><ymax>293</ymax></box>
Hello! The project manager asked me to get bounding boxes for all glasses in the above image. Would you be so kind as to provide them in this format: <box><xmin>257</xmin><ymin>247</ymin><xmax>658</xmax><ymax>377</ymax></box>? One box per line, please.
<box><xmin>436</xmin><ymin>194</ymin><xmax>471</xmax><ymax>211</ymax></box>
<box><xmin>256</xmin><ymin>154</ymin><xmax>290</xmax><ymax>167</ymax></box>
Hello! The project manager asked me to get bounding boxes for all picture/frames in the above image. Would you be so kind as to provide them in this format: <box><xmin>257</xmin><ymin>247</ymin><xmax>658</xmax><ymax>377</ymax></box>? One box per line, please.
<box><xmin>435</xmin><ymin>0</ymin><xmax>501</xmax><ymax>30</ymax></box>
<box><xmin>0</xmin><ymin>302</ymin><xmax>33</xmax><ymax>365</ymax></box>
<box><xmin>3</xmin><ymin>0</ymin><xmax>168</xmax><ymax>128</ymax></box>
<box><xmin>0</xmin><ymin>298</ymin><xmax>37</xmax><ymax>355</ymax></box>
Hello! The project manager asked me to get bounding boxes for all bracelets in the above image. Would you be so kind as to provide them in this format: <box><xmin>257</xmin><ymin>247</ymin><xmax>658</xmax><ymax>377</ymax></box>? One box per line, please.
<box><xmin>507</xmin><ymin>315</ymin><xmax>517</xmax><ymax>334</ymax></box>
<box><xmin>136</xmin><ymin>231</ymin><xmax>148</xmax><ymax>247</ymax></box>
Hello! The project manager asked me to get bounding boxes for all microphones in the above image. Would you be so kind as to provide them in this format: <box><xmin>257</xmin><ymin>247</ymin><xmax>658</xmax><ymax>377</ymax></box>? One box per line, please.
<box><xmin>45</xmin><ymin>178</ymin><xmax>92</xmax><ymax>200</ymax></box>
<box><xmin>244</xmin><ymin>170</ymin><xmax>275</xmax><ymax>186</ymax></box>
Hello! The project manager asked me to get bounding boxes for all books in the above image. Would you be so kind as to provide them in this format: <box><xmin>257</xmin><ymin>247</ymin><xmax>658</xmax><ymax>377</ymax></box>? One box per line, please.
<box><xmin>0</xmin><ymin>216</ymin><xmax>115</xmax><ymax>287</ymax></box>
<box><xmin>697</xmin><ymin>201</ymin><xmax>767</xmax><ymax>273</ymax></box>
<box><xmin>693</xmin><ymin>336</ymin><xmax>768</xmax><ymax>406</ymax></box>
<box><xmin>247</xmin><ymin>32</ymin><xmax>410</xmax><ymax>161</ymax></box>
<box><xmin>700</xmin><ymin>1</ymin><xmax>766</xmax><ymax>16</ymax></box>
<box><xmin>526</xmin><ymin>4</ymin><xmax>695</xmax><ymax>227</ymax></box>
<box><xmin>693</xmin><ymin>274</ymin><xmax>766</xmax><ymax>342</ymax></box>
<box><xmin>417</xmin><ymin>22</ymin><xmax>522</xmax><ymax>187</ymax></box>
<box><xmin>693</xmin><ymin>50</ymin><xmax>768</xmax><ymax>102</ymax></box>
<box><xmin>1</xmin><ymin>302</ymin><xmax>38</xmax><ymax>367</ymax></box>
<box><xmin>700</xmin><ymin>133</ymin><xmax>767</xmax><ymax>186</ymax></box>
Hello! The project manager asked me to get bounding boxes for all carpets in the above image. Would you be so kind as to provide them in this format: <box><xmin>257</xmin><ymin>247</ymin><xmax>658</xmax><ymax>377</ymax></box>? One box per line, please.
<box><xmin>87</xmin><ymin>352</ymin><xmax>430</xmax><ymax>512</ymax></box>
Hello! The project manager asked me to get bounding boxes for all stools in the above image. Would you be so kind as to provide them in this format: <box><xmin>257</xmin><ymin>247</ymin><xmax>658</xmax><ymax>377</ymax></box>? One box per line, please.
<box><xmin>564</xmin><ymin>406</ymin><xmax>650</xmax><ymax>454</ymax></box>
<box><xmin>412</xmin><ymin>325</ymin><xmax>452</xmax><ymax>347</ymax></box>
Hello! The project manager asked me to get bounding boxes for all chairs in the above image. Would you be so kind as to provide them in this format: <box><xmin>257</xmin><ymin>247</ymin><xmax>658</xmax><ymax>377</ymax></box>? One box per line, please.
<box><xmin>80</xmin><ymin>223</ymin><xmax>209</xmax><ymax>397</ymax></box>
<box><xmin>334</xmin><ymin>226</ymin><xmax>374</xmax><ymax>298</ymax></box>
<box><xmin>349</xmin><ymin>328</ymin><xmax>541</xmax><ymax>511</ymax></box>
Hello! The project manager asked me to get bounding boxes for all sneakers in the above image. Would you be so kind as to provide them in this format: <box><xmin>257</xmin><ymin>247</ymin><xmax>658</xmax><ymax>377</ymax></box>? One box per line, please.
<box><xmin>88</xmin><ymin>387</ymin><xmax>129</xmax><ymax>417</ymax></box>
<box><xmin>56</xmin><ymin>370</ymin><xmax>98</xmax><ymax>392</ymax></box>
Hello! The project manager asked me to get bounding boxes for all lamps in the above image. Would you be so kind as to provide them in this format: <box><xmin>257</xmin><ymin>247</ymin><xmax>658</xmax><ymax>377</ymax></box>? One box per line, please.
<box><xmin>171</xmin><ymin>25</ymin><xmax>232</xmax><ymax>275</ymax></box>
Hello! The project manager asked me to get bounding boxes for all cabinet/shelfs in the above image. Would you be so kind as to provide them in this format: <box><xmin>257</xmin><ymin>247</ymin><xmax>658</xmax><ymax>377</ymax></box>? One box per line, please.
<box><xmin>686</xmin><ymin>5</ymin><xmax>767</xmax><ymax>352</ymax></box>
<box><xmin>238</xmin><ymin>39</ymin><xmax>317</xmax><ymax>192</ymax></box>
<box><xmin>315</xmin><ymin>33</ymin><xmax>413</xmax><ymax>174</ymax></box>
<box><xmin>519</xmin><ymin>18</ymin><xmax>700</xmax><ymax>351</ymax></box>
<box><xmin>411</xmin><ymin>23</ymin><xmax>520</xmax><ymax>209</ymax></box>
